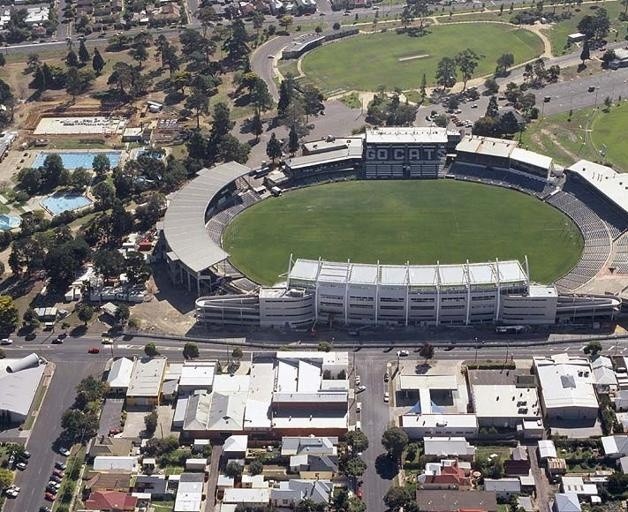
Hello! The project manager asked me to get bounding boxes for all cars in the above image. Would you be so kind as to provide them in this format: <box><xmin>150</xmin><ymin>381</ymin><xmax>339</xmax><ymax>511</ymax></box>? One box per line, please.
<box><xmin>1</xmin><ymin>337</ymin><xmax>115</xmax><ymax>354</ymax></box>
<box><xmin>450</xmin><ymin>97</ymin><xmax>507</xmax><ymax>129</ymax></box>
<box><xmin>6</xmin><ymin>450</ymin><xmax>31</xmax><ymax>498</ymax></box>
<box><xmin>38</xmin><ymin>447</ymin><xmax>71</xmax><ymax>512</ymax></box>
<box><xmin>352</xmin><ymin>348</ymin><xmax>413</xmax><ymax>413</ymax></box>
<box><xmin>589</xmin><ymin>86</ymin><xmax>595</xmax><ymax>92</ymax></box>
<box><xmin>543</xmin><ymin>96</ymin><xmax>551</xmax><ymax>102</ymax></box>
